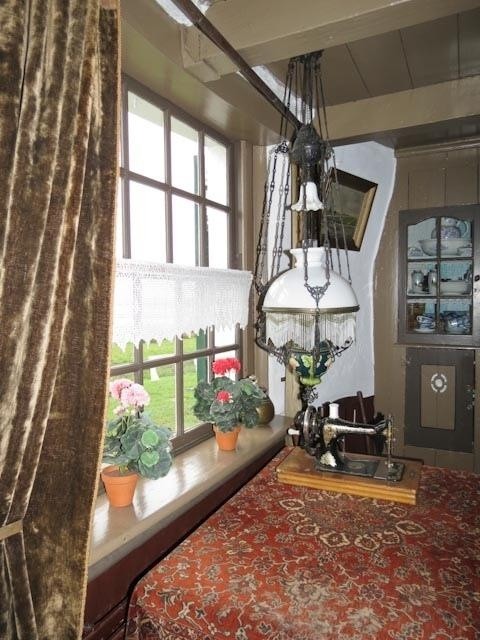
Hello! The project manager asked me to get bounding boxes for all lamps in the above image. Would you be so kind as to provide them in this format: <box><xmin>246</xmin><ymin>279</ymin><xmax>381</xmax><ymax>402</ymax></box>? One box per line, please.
<box><xmin>253</xmin><ymin>51</ymin><xmax>359</xmax><ymax>403</ymax></box>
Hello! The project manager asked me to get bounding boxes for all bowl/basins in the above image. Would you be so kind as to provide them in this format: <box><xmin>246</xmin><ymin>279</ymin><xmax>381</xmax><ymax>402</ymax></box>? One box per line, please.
<box><xmin>420</xmin><ymin>239</ymin><xmax>471</xmax><ymax>256</ymax></box>
<box><xmin>439</xmin><ymin>281</ymin><xmax>467</xmax><ymax>295</ymax></box>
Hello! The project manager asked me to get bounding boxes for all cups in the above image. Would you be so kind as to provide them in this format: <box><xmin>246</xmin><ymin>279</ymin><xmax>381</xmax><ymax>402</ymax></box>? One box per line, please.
<box><xmin>416</xmin><ymin>316</ymin><xmax>435</xmax><ymax>329</ymax></box>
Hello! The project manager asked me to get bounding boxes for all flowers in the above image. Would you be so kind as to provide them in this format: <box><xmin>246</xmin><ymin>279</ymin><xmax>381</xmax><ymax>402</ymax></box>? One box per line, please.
<box><xmin>193</xmin><ymin>357</ymin><xmax>259</xmax><ymax>430</ymax></box>
<box><xmin>100</xmin><ymin>380</ymin><xmax>172</xmax><ymax>479</ymax></box>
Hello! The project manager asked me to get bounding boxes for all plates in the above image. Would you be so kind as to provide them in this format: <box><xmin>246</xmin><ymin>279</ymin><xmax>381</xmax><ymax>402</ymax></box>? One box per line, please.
<box><xmin>413</xmin><ymin>329</ymin><xmax>435</xmax><ymax>333</ymax></box>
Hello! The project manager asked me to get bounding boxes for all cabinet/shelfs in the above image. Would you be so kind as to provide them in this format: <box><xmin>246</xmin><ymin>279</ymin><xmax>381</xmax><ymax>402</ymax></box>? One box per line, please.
<box><xmin>398</xmin><ymin>204</ymin><xmax>480</xmax><ymax>349</ymax></box>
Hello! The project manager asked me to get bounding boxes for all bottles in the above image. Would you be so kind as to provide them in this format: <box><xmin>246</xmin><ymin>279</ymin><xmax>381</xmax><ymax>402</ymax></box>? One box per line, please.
<box><xmin>256</xmin><ymin>386</ymin><xmax>274</xmax><ymax>425</ymax></box>
<box><xmin>411</xmin><ymin>268</ymin><xmax>437</xmax><ymax>294</ymax></box>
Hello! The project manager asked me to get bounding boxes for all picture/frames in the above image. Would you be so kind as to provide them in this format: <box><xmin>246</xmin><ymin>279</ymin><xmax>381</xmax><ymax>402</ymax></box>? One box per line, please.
<box><xmin>318</xmin><ymin>166</ymin><xmax>377</xmax><ymax>252</ymax></box>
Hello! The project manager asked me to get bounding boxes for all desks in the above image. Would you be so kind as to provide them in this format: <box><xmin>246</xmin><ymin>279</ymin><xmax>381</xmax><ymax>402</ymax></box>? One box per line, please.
<box><xmin>127</xmin><ymin>446</ymin><xmax>480</xmax><ymax>640</ymax></box>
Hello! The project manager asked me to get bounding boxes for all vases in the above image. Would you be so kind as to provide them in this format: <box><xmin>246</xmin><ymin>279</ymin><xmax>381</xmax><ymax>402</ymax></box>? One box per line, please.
<box><xmin>213</xmin><ymin>424</ymin><xmax>242</xmax><ymax>450</ymax></box>
<box><xmin>102</xmin><ymin>468</ymin><xmax>138</xmax><ymax>508</ymax></box>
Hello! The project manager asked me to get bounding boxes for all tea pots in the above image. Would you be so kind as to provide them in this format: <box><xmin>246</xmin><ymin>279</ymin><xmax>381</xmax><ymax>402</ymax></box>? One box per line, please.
<box><xmin>429</xmin><ymin>218</ymin><xmax>468</xmax><ymax>239</ymax></box>
